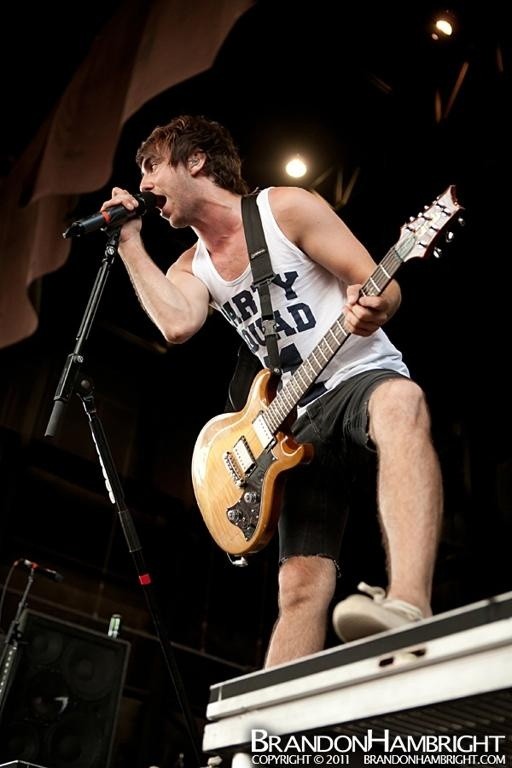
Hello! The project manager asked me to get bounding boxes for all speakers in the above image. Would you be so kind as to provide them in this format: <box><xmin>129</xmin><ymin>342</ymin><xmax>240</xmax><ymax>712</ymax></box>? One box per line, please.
<box><xmin>0</xmin><ymin>607</ymin><xmax>134</xmax><ymax>768</ymax></box>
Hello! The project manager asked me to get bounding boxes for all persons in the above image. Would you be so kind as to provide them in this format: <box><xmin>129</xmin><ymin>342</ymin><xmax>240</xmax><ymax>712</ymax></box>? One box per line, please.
<box><xmin>99</xmin><ymin>115</ymin><xmax>445</xmax><ymax>671</ymax></box>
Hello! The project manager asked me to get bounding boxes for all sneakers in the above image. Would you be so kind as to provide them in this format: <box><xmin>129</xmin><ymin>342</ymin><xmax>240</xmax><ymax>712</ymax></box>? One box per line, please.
<box><xmin>332</xmin><ymin>580</ymin><xmax>424</xmax><ymax>643</ymax></box>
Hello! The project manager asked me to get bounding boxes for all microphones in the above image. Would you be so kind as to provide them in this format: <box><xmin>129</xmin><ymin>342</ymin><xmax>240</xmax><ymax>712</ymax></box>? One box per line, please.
<box><xmin>20</xmin><ymin>557</ymin><xmax>64</xmax><ymax>583</ymax></box>
<box><xmin>62</xmin><ymin>190</ymin><xmax>159</xmax><ymax>241</ymax></box>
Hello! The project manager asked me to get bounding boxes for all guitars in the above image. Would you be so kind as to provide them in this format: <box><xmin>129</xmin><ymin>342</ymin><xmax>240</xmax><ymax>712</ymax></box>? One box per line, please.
<box><xmin>191</xmin><ymin>183</ymin><xmax>467</xmax><ymax>568</ymax></box>
